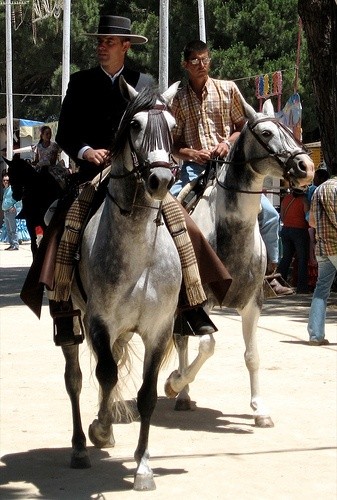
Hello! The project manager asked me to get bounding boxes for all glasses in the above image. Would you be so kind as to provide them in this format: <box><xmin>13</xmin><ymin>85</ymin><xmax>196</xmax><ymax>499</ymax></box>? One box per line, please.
<box><xmin>187</xmin><ymin>58</ymin><xmax>211</xmax><ymax>64</ymax></box>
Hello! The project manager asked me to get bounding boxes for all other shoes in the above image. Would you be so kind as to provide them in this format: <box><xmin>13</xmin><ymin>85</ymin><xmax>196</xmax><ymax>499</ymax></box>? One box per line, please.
<box><xmin>57</xmin><ymin>328</ymin><xmax>76</xmax><ymax>346</ymax></box>
<box><xmin>309</xmin><ymin>339</ymin><xmax>329</xmax><ymax>345</ymax></box>
<box><xmin>191</xmin><ymin>320</ymin><xmax>215</xmax><ymax>334</ymax></box>
<box><xmin>269</xmin><ymin>286</ymin><xmax>293</xmax><ymax>295</ymax></box>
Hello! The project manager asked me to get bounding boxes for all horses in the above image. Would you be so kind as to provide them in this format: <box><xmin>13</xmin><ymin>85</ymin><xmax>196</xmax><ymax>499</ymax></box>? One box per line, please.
<box><xmin>164</xmin><ymin>93</ymin><xmax>316</xmax><ymax>428</ymax></box>
<box><xmin>48</xmin><ymin>75</ymin><xmax>184</xmax><ymax>491</ymax></box>
<box><xmin>1</xmin><ymin>152</ymin><xmax>71</xmax><ymax>262</ymax></box>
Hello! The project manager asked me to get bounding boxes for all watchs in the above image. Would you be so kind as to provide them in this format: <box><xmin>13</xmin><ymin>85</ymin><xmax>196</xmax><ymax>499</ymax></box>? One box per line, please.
<box><xmin>221</xmin><ymin>140</ymin><xmax>233</xmax><ymax>150</ymax></box>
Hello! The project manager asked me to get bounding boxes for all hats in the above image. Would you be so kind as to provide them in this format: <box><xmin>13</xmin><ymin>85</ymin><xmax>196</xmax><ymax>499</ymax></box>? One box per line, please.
<box><xmin>82</xmin><ymin>15</ymin><xmax>148</xmax><ymax>44</ymax></box>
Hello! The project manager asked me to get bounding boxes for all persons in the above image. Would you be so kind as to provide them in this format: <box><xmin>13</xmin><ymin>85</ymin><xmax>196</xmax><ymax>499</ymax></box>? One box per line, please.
<box><xmin>48</xmin><ymin>17</ymin><xmax>217</xmax><ymax>345</ymax></box>
<box><xmin>277</xmin><ymin>170</ymin><xmax>337</xmax><ymax>346</ymax></box>
<box><xmin>167</xmin><ymin>40</ymin><xmax>296</xmax><ymax>298</ymax></box>
<box><xmin>0</xmin><ymin>126</ymin><xmax>73</xmax><ymax>251</ymax></box>
